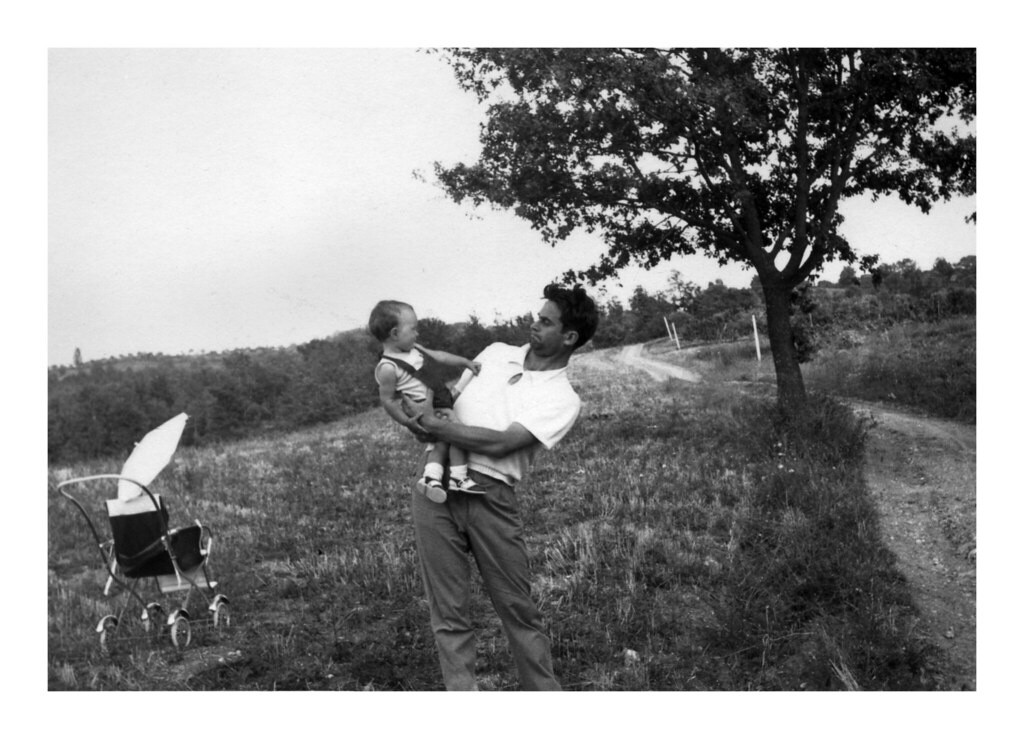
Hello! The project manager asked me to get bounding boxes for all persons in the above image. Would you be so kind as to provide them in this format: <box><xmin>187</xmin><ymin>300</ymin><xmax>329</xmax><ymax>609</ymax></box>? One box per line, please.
<box><xmin>368</xmin><ymin>301</ymin><xmax>488</xmax><ymax>502</ymax></box>
<box><xmin>411</xmin><ymin>284</ymin><xmax>600</xmax><ymax>691</ymax></box>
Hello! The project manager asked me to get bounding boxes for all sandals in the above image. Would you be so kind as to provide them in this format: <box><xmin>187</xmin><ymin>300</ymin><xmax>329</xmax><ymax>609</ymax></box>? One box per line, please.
<box><xmin>448</xmin><ymin>477</ymin><xmax>487</xmax><ymax>494</ymax></box>
<box><xmin>416</xmin><ymin>477</ymin><xmax>447</xmax><ymax>502</ymax></box>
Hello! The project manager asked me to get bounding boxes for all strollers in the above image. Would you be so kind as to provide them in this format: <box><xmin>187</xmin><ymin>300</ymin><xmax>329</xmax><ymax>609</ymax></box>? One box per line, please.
<box><xmin>56</xmin><ymin>474</ymin><xmax>231</xmax><ymax>655</ymax></box>
